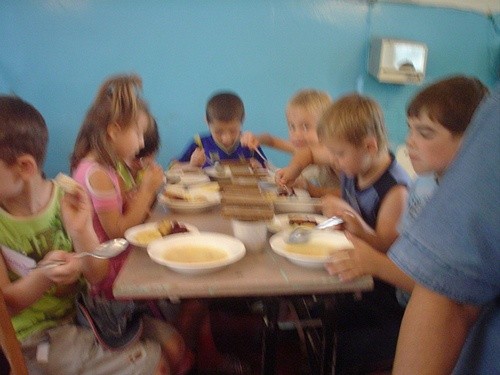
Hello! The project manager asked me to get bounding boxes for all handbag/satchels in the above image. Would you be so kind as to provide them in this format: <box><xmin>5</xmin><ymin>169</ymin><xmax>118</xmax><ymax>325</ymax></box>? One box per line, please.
<box><xmin>74</xmin><ymin>290</ymin><xmax>148</xmax><ymax>350</ymax></box>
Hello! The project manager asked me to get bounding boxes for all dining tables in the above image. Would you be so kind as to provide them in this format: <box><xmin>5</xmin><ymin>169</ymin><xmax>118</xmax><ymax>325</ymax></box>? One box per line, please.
<box><xmin>111</xmin><ymin>169</ymin><xmax>373</xmax><ymax>375</ymax></box>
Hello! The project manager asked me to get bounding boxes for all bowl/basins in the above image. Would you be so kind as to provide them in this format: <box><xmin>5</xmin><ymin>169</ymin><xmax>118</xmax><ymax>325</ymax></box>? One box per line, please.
<box><xmin>147</xmin><ymin>232</ymin><xmax>246</xmax><ymax>275</ymax></box>
<box><xmin>270</xmin><ymin>229</ymin><xmax>353</xmax><ymax>268</ymax></box>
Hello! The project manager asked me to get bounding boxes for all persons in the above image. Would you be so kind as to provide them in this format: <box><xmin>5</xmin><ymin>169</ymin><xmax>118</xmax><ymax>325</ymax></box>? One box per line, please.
<box><xmin>175</xmin><ymin>91</ymin><xmax>268</xmax><ymax>170</ymax></box>
<box><xmin>0</xmin><ymin>94</ymin><xmax>190</xmax><ymax>375</ymax></box>
<box><xmin>71</xmin><ymin>77</ymin><xmax>252</xmax><ymax>375</ymax></box>
<box><xmin>127</xmin><ymin>113</ymin><xmax>162</xmax><ymax>206</ymax></box>
<box><xmin>386</xmin><ymin>85</ymin><xmax>500</xmax><ymax>375</ymax></box>
<box><xmin>321</xmin><ymin>77</ymin><xmax>486</xmax><ymax>375</ymax></box>
<box><xmin>238</xmin><ymin>87</ymin><xmax>342</xmax><ymax>329</ymax></box>
<box><xmin>273</xmin><ymin>93</ymin><xmax>415</xmax><ymax>253</ymax></box>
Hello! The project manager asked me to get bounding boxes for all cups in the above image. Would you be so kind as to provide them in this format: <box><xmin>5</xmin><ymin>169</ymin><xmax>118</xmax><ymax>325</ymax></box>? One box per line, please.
<box><xmin>233</xmin><ymin>218</ymin><xmax>266</xmax><ymax>251</ymax></box>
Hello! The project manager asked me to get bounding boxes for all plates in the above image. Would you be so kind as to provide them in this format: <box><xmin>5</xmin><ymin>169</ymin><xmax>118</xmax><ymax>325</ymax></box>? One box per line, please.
<box><xmin>125</xmin><ymin>222</ymin><xmax>199</xmax><ymax>248</ymax></box>
<box><xmin>267</xmin><ymin>212</ymin><xmax>327</xmax><ymax>233</ymax></box>
<box><xmin>158</xmin><ymin>192</ymin><xmax>220</xmax><ymax>211</ymax></box>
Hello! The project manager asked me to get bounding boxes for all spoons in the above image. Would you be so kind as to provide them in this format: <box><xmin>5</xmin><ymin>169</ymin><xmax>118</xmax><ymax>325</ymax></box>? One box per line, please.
<box><xmin>25</xmin><ymin>238</ymin><xmax>127</xmax><ymax>271</ymax></box>
<box><xmin>286</xmin><ymin>216</ymin><xmax>344</xmax><ymax>244</ymax></box>
